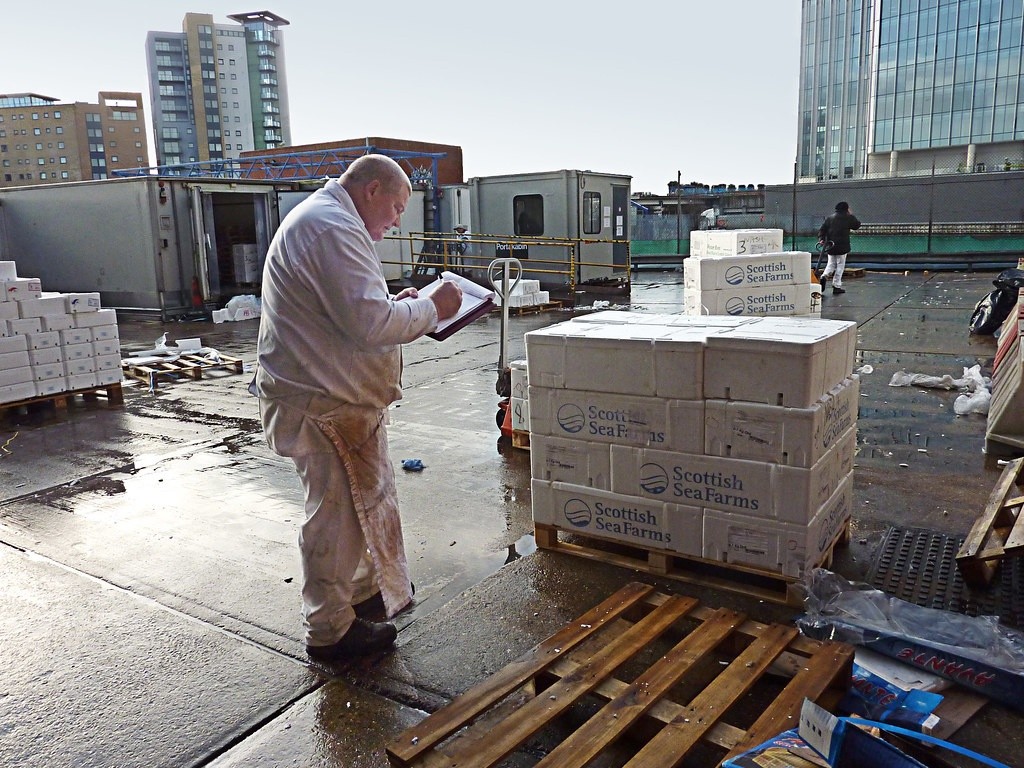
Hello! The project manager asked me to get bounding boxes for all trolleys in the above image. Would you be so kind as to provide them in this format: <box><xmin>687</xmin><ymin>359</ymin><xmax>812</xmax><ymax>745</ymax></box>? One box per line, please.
<box><xmin>811</xmin><ymin>241</ymin><xmax>835</xmax><ymax>291</ymax></box>
<box><xmin>488</xmin><ymin>258</ymin><xmax>531</xmax><ymax>450</ymax></box>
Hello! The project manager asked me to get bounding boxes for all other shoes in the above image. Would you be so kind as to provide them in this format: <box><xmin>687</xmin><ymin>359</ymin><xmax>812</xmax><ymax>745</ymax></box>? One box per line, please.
<box><xmin>833</xmin><ymin>287</ymin><xmax>846</xmax><ymax>294</ymax></box>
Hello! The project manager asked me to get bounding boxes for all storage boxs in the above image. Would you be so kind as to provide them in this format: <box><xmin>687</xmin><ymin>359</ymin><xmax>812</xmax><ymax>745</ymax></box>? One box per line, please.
<box><xmin>0</xmin><ymin>260</ymin><xmax>124</xmax><ymax>404</ymax></box>
<box><xmin>232</xmin><ymin>243</ymin><xmax>258</xmax><ymax>283</ymax></box>
<box><xmin>492</xmin><ymin>228</ymin><xmax>860</xmax><ymax>579</ymax></box>
<box><xmin>850</xmin><ymin>645</ymin><xmax>990</xmax><ymax>749</ymax></box>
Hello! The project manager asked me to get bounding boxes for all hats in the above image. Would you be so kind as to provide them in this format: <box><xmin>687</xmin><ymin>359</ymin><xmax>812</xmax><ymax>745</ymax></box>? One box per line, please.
<box><xmin>835</xmin><ymin>202</ymin><xmax>848</xmax><ymax>213</ymax></box>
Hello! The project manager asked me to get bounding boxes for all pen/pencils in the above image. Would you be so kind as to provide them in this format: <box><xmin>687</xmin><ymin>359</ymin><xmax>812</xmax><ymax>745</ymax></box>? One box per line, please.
<box><xmin>436</xmin><ymin>267</ymin><xmax>444</xmax><ymax>283</ymax></box>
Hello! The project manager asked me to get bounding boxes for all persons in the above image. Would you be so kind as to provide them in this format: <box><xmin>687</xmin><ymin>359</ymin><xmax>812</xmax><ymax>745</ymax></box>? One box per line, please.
<box><xmin>453</xmin><ymin>224</ymin><xmax>473</xmax><ymax>276</ymax></box>
<box><xmin>818</xmin><ymin>202</ymin><xmax>861</xmax><ymax>293</ymax></box>
<box><xmin>247</xmin><ymin>154</ymin><xmax>462</xmax><ymax>657</ymax></box>
<box><xmin>700</xmin><ymin>203</ymin><xmax>720</xmax><ymax>230</ymax></box>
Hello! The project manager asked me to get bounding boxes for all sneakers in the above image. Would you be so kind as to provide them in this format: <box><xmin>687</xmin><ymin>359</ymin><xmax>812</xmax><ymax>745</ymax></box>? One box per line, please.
<box><xmin>352</xmin><ymin>581</ymin><xmax>415</xmax><ymax>615</ymax></box>
<box><xmin>306</xmin><ymin>618</ymin><xmax>398</xmax><ymax>655</ymax></box>
